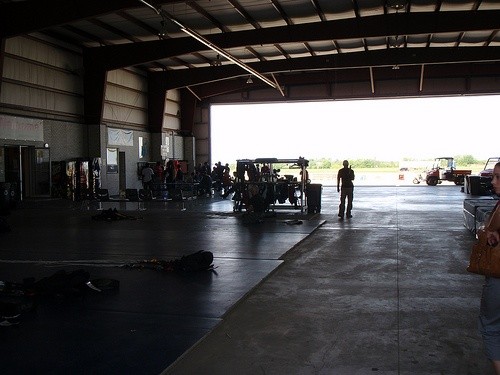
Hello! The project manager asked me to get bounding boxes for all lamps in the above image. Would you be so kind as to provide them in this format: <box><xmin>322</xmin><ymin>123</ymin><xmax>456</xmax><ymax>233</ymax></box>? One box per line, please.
<box><xmin>213</xmin><ymin>54</ymin><xmax>222</xmax><ymax>66</ymax></box>
<box><xmin>247</xmin><ymin>75</ymin><xmax>253</xmax><ymax>83</ymax></box>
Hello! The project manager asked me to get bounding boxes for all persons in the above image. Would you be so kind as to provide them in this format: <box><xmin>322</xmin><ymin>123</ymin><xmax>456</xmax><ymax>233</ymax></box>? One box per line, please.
<box><xmin>337</xmin><ymin>161</ymin><xmax>355</xmax><ymax>218</ymax></box>
<box><xmin>478</xmin><ymin>163</ymin><xmax>500</xmax><ymax>375</ymax></box>
<box><xmin>139</xmin><ymin>158</ymin><xmax>308</xmax><ymax>201</ymax></box>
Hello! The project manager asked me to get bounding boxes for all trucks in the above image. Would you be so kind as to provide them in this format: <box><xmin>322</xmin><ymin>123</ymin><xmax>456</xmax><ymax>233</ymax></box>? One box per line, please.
<box><xmin>426</xmin><ymin>157</ymin><xmax>472</xmax><ymax>187</ymax></box>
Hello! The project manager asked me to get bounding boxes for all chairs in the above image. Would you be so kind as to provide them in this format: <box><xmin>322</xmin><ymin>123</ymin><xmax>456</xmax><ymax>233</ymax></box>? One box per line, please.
<box><xmin>80</xmin><ymin>188</ymin><xmax>157</xmax><ymax>212</ymax></box>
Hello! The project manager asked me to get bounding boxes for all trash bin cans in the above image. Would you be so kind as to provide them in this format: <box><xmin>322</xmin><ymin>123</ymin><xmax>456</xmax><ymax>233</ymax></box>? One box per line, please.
<box><xmin>304</xmin><ymin>184</ymin><xmax>323</xmax><ymax>215</ymax></box>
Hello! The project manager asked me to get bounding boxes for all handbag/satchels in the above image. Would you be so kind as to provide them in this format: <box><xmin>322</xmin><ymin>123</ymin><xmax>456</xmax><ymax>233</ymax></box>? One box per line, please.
<box><xmin>468</xmin><ymin>229</ymin><xmax>500</xmax><ymax>278</ymax></box>
